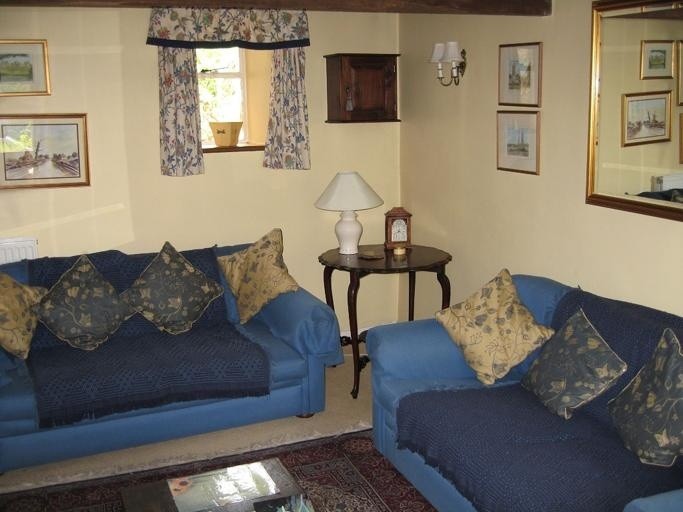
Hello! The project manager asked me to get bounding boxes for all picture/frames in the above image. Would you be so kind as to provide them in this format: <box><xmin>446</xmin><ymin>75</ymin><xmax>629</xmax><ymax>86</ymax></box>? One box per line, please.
<box><xmin>0</xmin><ymin>110</ymin><xmax>91</xmax><ymax>190</ymax></box>
<box><xmin>619</xmin><ymin>88</ymin><xmax>672</xmax><ymax>147</ymax></box>
<box><xmin>495</xmin><ymin>40</ymin><xmax>543</xmax><ymax>108</ymax></box>
<box><xmin>495</xmin><ymin>110</ymin><xmax>543</xmax><ymax>178</ymax></box>
<box><xmin>0</xmin><ymin>38</ymin><xmax>52</xmax><ymax>101</ymax></box>
<box><xmin>637</xmin><ymin>39</ymin><xmax>676</xmax><ymax>83</ymax></box>
<box><xmin>674</xmin><ymin>39</ymin><xmax>683</xmax><ymax>109</ymax></box>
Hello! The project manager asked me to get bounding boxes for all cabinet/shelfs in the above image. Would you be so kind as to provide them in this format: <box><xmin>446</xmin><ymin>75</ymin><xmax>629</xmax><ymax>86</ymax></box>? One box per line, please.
<box><xmin>322</xmin><ymin>51</ymin><xmax>402</xmax><ymax>125</ymax></box>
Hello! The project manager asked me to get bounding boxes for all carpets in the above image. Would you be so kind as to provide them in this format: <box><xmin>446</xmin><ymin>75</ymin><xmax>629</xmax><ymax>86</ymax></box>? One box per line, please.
<box><xmin>0</xmin><ymin>419</ymin><xmax>440</xmax><ymax>512</ymax></box>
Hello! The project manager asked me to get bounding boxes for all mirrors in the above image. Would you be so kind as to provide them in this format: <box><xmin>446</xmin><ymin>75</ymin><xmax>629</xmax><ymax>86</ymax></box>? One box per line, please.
<box><xmin>583</xmin><ymin>0</ymin><xmax>682</xmax><ymax>222</ymax></box>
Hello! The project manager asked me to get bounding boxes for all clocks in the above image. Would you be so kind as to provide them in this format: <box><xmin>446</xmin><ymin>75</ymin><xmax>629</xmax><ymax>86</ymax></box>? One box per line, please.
<box><xmin>382</xmin><ymin>205</ymin><xmax>414</xmax><ymax>253</ymax></box>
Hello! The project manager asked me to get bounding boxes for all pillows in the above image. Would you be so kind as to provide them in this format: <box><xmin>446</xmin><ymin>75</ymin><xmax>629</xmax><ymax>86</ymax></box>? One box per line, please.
<box><xmin>0</xmin><ymin>268</ymin><xmax>51</xmax><ymax>366</ymax></box>
<box><xmin>602</xmin><ymin>323</ymin><xmax>682</xmax><ymax>468</ymax></box>
<box><xmin>669</xmin><ymin>189</ymin><xmax>682</xmax><ymax>204</ymax></box>
<box><xmin>24</xmin><ymin>249</ymin><xmax>142</xmax><ymax>353</ymax></box>
<box><xmin>214</xmin><ymin>225</ymin><xmax>300</xmax><ymax>328</ymax></box>
<box><xmin>437</xmin><ymin>267</ymin><xmax>559</xmax><ymax>389</ymax></box>
<box><xmin>116</xmin><ymin>239</ymin><xmax>224</xmax><ymax>337</ymax></box>
<box><xmin>521</xmin><ymin>306</ymin><xmax>630</xmax><ymax>425</ymax></box>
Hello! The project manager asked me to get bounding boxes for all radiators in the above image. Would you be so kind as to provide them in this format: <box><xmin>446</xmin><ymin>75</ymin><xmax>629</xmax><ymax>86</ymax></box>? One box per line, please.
<box><xmin>0</xmin><ymin>235</ymin><xmax>41</xmax><ymax>267</ymax></box>
<box><xmin>648</xmin><ymin>171</ymin><xmax>682</xmax><ymax>195</ymax></box>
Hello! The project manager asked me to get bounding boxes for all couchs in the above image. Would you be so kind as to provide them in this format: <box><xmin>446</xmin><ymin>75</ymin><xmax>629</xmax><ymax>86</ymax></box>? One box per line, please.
<box><xmin>364</xmin><ymin>273</ymin><xmax>682</xmax><ymax>512</ymax></box>
<box><xmin>0</xmin><ymin>240</ymin><xmax>346</xmax><ymax>477</ymax></box>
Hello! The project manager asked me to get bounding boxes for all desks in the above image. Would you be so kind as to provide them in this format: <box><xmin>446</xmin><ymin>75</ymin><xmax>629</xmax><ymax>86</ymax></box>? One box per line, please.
<box><xmin>314</xmin><ymin>243</ymin><xmax>452</xmax><ymax>403</ymax></box>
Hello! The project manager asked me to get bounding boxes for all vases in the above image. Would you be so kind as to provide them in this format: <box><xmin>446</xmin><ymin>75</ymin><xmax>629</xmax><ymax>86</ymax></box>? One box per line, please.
<box><xmin>208</xmin><ymin>120</ymin><xmax>244</xmax><ymax>148</ymax></box>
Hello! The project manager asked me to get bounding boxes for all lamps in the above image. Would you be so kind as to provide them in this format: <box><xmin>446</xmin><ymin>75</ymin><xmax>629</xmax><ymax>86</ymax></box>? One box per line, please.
<box><xmin>311</xmin><ymin>170</ymin><xmax>383</xmax><ymax>257</ymax></box>
<box><xmin>429</xmin><ymin>38</ymin><xmax>467</xmax><ymax>88</ymax></box>
